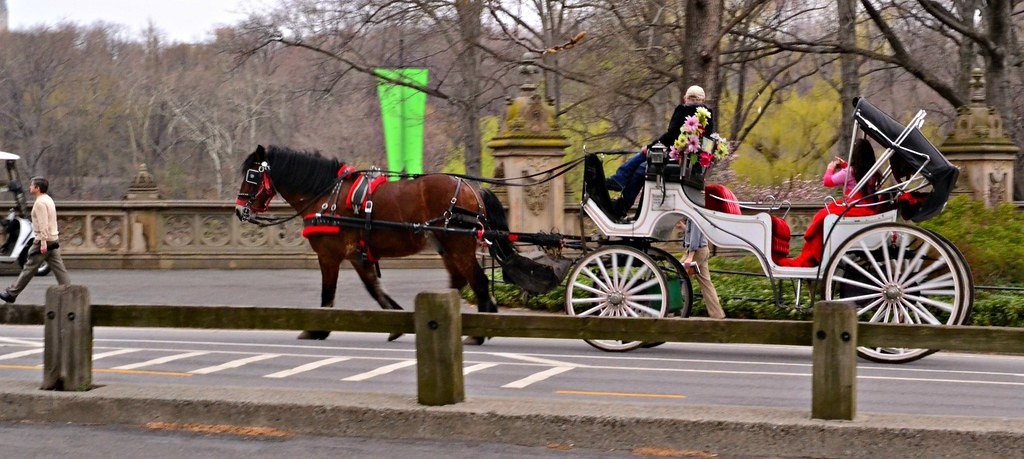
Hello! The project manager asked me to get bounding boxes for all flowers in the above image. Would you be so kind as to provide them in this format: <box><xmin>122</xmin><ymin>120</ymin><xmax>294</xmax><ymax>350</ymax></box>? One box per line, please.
<box><xmin>709</xmin><ymin>131</ymin><xmax>730</xmax><ymax>163</ymax></box>
<box><xmin>668</xmin><ymin>106</ymin><xmax>712</xmax><ymax>162</ymax></box>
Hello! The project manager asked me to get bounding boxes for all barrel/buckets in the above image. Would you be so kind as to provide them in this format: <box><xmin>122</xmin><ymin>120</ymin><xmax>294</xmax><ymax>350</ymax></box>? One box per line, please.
<box><xmin>648</xmin><ymin>261</ymin><xmax>684</xmax><ymax>312</ymax></box>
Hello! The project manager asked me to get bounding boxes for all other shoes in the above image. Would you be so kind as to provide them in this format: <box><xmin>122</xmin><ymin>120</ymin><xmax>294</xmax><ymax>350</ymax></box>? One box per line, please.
<box><xmin>605</xmin><ymin>177</ymin><xmax>621</xmax><ymax>192</ymax></box>
<box><xmin>0</xmin><ymin>291</ymin><xmax>15</xmax><ymax>303</ymax></box>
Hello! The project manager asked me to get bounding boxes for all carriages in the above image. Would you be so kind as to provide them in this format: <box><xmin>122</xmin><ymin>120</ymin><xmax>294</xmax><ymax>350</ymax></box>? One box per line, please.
<box><xmin>236</xmin><ymin>97</ymin><xmax>974</xmax><ymax>364</ymax></box>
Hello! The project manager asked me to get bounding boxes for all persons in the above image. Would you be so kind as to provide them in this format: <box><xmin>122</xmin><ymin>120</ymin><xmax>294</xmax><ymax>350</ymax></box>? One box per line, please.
<box><xmin>676</xmin><ymin>217</ymin><xmax>726</xmax><ymax>319</ymax></box>
<box><xmin>0</xmin><ymin>176</ymin><xmax>71</xmax><ymax>303</ymax></box>
<box><xmin>606</xmin><ymin>86</ymin><xmax>713</xmax><ymax>217</ymax></box>
<box><xmin>804</xmin><ymin>138</ymin><xmax>883</xmax><ymax>265</ymax></box>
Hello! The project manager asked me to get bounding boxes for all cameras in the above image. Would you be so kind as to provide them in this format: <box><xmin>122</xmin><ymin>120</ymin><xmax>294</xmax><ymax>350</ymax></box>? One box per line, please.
<box><xmin>834</xmin><ymin>163</ymin><xmax>841</xmax><ymax>169</ymax></box>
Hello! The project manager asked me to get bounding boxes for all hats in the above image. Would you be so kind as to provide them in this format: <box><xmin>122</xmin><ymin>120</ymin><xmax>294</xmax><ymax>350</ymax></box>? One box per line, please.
<box><xmin>686</xmin><ymin>86</ymin><xmax>706</xmax><ymax>99</ymax></box>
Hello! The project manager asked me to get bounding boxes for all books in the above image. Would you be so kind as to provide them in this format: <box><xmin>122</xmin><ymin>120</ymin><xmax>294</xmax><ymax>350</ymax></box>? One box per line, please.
<box><xmin>681</xmin><ymin>261</ymin><xmax>700</xmax><ymax>274</ymax></box>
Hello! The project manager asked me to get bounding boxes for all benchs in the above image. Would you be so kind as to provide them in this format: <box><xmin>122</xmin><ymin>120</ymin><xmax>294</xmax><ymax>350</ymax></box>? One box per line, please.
<box><xmin>820</xmin><ymin>188</ymin><xmax>896</xmax><ymax>269</ymax></box>
<box><xmin>647</xmin><ymin>129</ymin><xmax>718</xmax><ymax>191</ymax></box>
<box><xmin>705</xmin><ymin>183</ymin><xmax>791</xmax><ymax>257</ymax></box>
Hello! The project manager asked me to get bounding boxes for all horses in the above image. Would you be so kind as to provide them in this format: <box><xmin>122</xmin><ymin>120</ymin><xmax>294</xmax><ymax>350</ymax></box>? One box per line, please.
<box><xmin>235</xmin><ymin>144</ymin><xmax>549</xmax><ymax>344</ymax></box>
